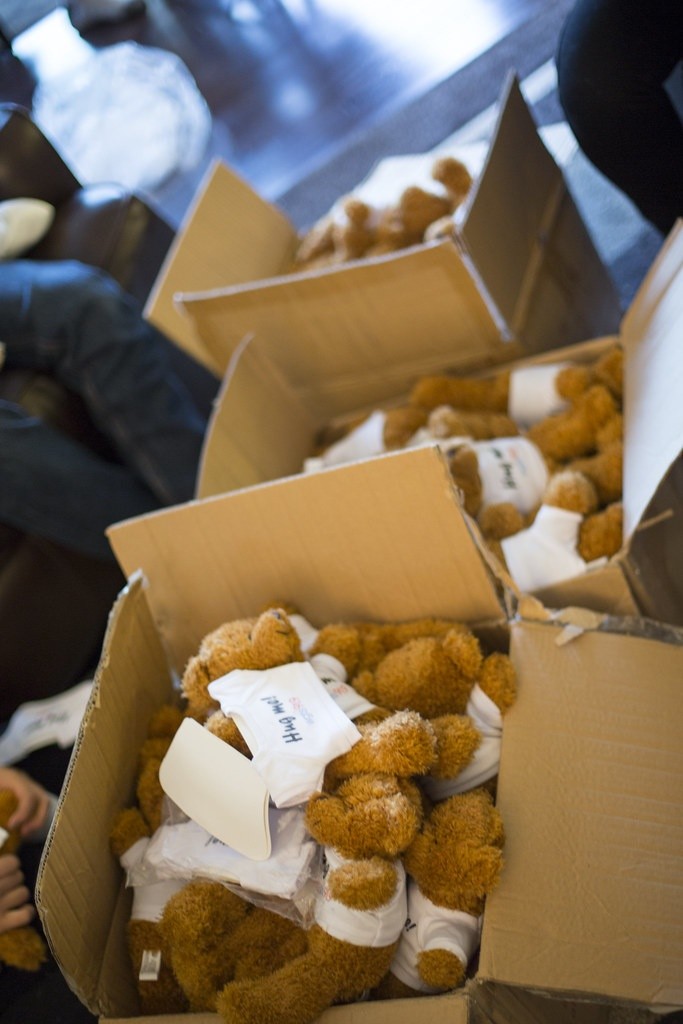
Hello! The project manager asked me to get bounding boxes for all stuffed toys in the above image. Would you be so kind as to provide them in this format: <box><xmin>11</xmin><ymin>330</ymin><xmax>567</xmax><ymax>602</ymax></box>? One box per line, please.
<box><xmin>279</xmin><ymin>156</ymin><xmax>476</xmax><ymax>276</ymax></box>
<box><xmin>297</xmin><ymin>346</ymin><xmax>623</xmax><ymax>593</ymax></box>
<box><xmin>0</xmin><ymin>786</ymin><xmax>47</xmax><ymax>970</ymax></box>
<box><xmin>109</xmin><ymin>594</ymin><xmax>519</xmax><ymax>1024</ymax></box>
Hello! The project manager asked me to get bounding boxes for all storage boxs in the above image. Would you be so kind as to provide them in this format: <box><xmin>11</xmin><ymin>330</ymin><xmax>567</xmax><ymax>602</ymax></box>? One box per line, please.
<box><xmin>142</xmin><ymin>70</ymin><xmax>620</xmax><ymax>400</ymax></box>
<box><xmin>196</xmin><ymin>217</ymin><xmax>683</xmax><ymax>624</ymax></box>
<box><xmin>35</xmin><ymin>444</ymin><xmax>683</xmax><ymax>1024</ymax></box>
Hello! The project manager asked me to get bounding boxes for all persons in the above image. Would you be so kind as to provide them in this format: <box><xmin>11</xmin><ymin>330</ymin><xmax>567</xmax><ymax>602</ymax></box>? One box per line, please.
<box><xmin>555</xmin><ymin>1</ymin><xmax>683</xmax><ymax>239</ymax></box>
<box><xmin>0</xmin><ymin>741</ymin><xmax>101</xmax><ymax>1024</ymax></box>
<box><xmin>1</xmin><ymin>261</ymin><xmax>209</xmax><ymax>561</ymax></box>
